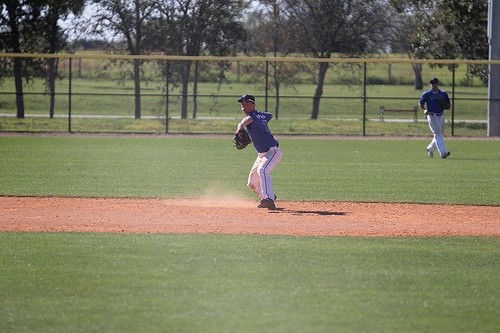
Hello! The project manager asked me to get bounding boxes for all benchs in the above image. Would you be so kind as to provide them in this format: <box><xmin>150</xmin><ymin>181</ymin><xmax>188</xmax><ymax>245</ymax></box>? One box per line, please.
<box><xmin>380</xmin><ymin>105</ymin><xmax>418</xmax><ymax>123</ymax></box>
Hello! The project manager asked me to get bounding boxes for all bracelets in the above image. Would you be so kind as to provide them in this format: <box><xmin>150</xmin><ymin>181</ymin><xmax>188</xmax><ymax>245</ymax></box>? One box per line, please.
<box><xmin>239</xmin><ymin>122</ymin><xmax>244</xmax><ymax>127</ymax></box>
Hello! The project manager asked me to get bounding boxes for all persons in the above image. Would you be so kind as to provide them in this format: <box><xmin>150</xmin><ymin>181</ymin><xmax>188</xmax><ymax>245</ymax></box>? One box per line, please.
<box><xmin>420</xmin><ymin>78</ymin><xmax>451</xmax><ymax>159</ymax></box>
<box><xmin>232</xmin><ymin>94</ymin><xmax>282</xmax><ymax>210</ymax></box>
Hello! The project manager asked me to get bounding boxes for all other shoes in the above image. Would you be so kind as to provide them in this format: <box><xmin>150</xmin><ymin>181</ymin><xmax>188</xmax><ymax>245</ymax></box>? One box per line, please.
<box><xmin>258</xmin><ymin>194</ymin><xmax>277</xmax><ymax>210</ymax></box>
<box><xmin>426</xmin><ymin>148</ymin><xmax>434</xmax><ymax>158</ymax></box>
<box><xmin>442</xmin><ymin>151</ymin><xmax>450</xmax><ymax>158</ymax></box>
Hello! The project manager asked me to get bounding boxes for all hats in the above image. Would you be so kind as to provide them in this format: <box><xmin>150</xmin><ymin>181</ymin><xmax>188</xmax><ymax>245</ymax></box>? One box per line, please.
<box><xmin>430</xmin><ymin>78</ymin><xmax>438</xmax><ymax>83</ymax></box>
<box><xmin>238</xmin><ymin>95</ymin><xmax>255</xmax><ymax>104</ymax></box>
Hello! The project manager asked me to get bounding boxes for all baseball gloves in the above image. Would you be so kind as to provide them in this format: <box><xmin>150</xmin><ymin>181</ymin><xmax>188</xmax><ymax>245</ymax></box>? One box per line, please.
<box><xmin>234</xmin><ymin>129</ymin><xmax>251</xmax><ymax>150</ymax></box>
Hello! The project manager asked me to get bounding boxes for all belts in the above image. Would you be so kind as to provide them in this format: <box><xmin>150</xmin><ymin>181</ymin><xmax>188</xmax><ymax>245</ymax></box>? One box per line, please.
<box><xmin>431</xmin><ymin>113</ymin><xmax>442</xmax><ymax>116</ymax></box>
